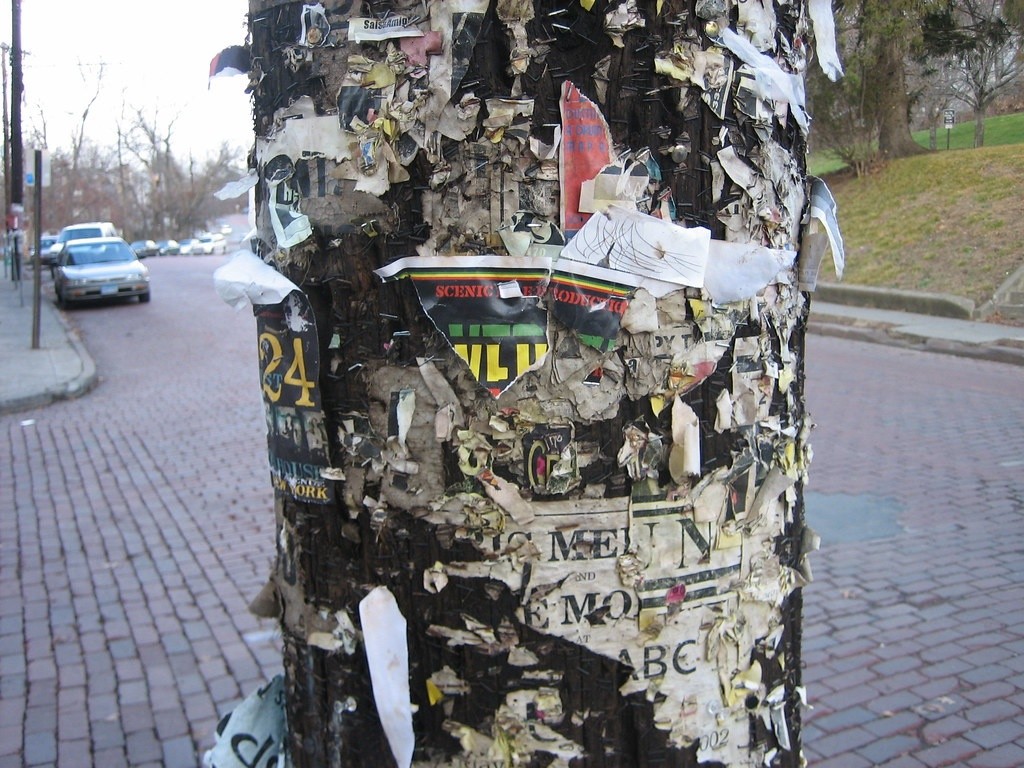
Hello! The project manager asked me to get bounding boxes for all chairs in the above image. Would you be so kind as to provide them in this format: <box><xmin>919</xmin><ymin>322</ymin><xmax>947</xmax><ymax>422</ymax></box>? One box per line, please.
<box><xmin>105</xmin><ymin>248</ymin><xmax>116</xmax><ymax>258</ymax></box>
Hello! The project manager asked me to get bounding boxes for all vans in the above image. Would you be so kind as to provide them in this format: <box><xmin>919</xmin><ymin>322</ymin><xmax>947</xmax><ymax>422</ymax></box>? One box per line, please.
<box><xmin>49</xmin><ymin>222</ymin><xmax>118</xmax><ymax>278</ymax></box>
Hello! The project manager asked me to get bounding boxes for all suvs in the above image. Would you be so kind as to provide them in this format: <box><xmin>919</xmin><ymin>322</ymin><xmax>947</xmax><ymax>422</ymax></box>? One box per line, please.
<box><xmin>201</xmin><ymin>234</ymin><xmax>227</xmax><ymax>253</ymax></box>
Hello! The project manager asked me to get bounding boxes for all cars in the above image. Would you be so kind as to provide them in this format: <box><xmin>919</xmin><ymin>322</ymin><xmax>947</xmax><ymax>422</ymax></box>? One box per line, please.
<box><xmin>130</xmin><ymin>239</ymin><xmax>157</xmax><ymax>259</ymax></box>
<box><xmin>154</xmin><ymin>241</ymin><xmax>179</xmax><ymax>256</ymax></box>
<box><xmin>55</xmin><ymin>237</ymin><xmax>150</xmax><ymax>309</ymax></box>
<box><xmin>180</xmin><ymin>238</ymin><xmax>203</xmax><ymax>255</ymax></box>
